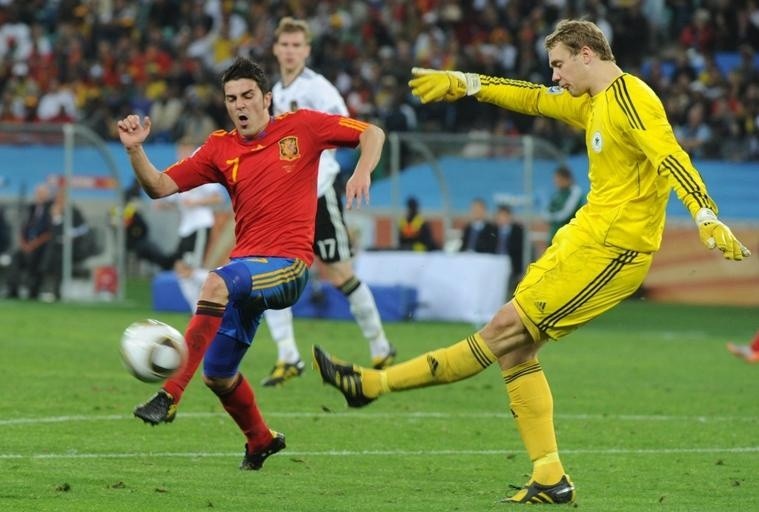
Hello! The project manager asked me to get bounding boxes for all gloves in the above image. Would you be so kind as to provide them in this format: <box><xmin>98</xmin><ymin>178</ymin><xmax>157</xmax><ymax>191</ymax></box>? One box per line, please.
<box><xmin>693</xmin><ymin>207</ymin><xmax>751</xmax><ymax>262</ymax></box>
<box><xmin>408</xmin><ymin>68</ymin><xmax>482</xmax><ymax>105</ymax></box>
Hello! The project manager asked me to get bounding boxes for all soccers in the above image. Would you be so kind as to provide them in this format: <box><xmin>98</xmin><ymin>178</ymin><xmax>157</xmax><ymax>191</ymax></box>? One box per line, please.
<box><xmin>120</xmin><ymin>320</ymin><xmax>188</xmax><ymax>383</ymax></box>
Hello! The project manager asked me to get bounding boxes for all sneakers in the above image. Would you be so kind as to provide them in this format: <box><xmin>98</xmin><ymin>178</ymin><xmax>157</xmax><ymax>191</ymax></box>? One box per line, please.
<box><xmin>311</xmin><ymin>344</ymin><xmax>377</xmax><ymax>408</ymax></box>
<box><xmin>132</xmin><ymin>387</ymin><xmax>178</xmax><ymax>427</ymax></box>
<box><xmin>261</xmin><ymin>357</ymin><xmax>305</xmax><ymax>387</ymax></box>
<box><xmin>372</xmin><ymin>343</ymin><xmax>396</xmax><ymax>370</ymax></box>
<box><xmin>495</xmin><ymin>472</ymin><xmax>578</xmax><ymax>504</ymax></box>
<box><xmin>239</xmin><ymin>430</ymin><xmax>286</xmax><ymax>469</ymax></box>
<box><xmin>725</xmin><ymin>343</ymin><xmax>758</xmax><ymax>366</ymax></box>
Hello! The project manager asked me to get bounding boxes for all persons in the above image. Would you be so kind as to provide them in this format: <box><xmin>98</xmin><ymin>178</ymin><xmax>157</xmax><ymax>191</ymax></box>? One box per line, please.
<box><xmin>262</xmin><ymin>17</ymin><xmax>394</xmax><ymax>387</ymax></box>
<box><xmin>312</xmin><ymin>18</ymin><xmax>751</xmax><ymax>506</ymax></box>
<box><xmin>116</xmin><ymin>57</ymin><xmax>387</xmax><ymax>470</ymax></box>
<box><xmin>1</xmin><ymin>1</ymin><xmax>758</xmax><ymax>316</ymax></box>
